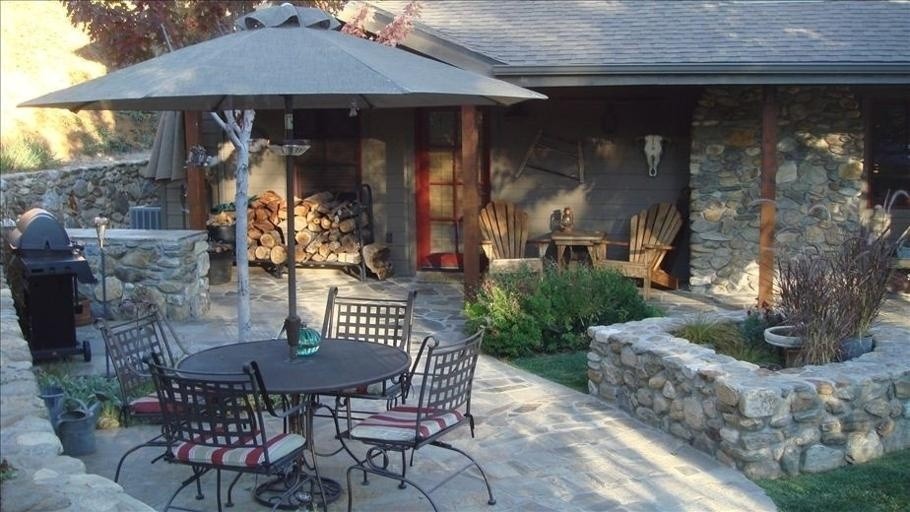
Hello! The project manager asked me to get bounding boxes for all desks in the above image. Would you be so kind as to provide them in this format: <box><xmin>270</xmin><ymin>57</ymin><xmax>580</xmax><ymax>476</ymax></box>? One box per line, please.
<box><xmin>553</xmin><ymin>229</ymin><xmax>605</xmax><ymax>269</ymax></box>
<box><xmin>173</xmin><ymin>337</ymin><xmax>413</xmax><ymax>511</ymax></box>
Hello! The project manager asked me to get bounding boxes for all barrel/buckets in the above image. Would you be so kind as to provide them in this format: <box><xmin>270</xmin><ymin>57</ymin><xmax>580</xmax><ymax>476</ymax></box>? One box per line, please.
<box><xmin>53</xmin><ymin>408</ymin><xmax>97</xmax><ymax>455</ymax></box>
<box><xmin>208</xmin><ymin>249</ymin><xmax>234</xmax><ymax>285</ymax></box>
<box><xmin>207</xmin><ymin>221</ymin><xmax>236</xmax><ymax>244</ymax></box>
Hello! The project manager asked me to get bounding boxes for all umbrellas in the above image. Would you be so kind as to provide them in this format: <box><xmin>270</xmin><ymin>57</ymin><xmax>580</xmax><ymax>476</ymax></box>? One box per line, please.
<box><xmin>15</xmin><ymin>4</ymin><xmax>550</xmax><ymax>480</ymax></box>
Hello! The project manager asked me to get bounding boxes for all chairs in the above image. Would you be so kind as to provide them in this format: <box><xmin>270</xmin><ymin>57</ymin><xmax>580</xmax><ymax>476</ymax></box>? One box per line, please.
<box><xmin>96</xmin><ymin>303</ymin><xmax>256</xmax><ymax>500</ymax></box>
<box><xmin>589</xmin><ymin>201</ymin><xmax>684</xmax><ymax>301</ymax></box>
<box><xmin>478</xmin><ymin>199</ymin><xmax>552</xmax><ymax>284</ymax></box>
<box><xmin>145</xmin><ymin>359</ymin><xmax>328</xmax><ymax>511</ymax></box>
<box><xmin>302</xmin><ymin>288</ymin><xmax>416</xmax><ymax>456</ymax></box>
<box><xmin>335</xmin><ymin>325</ymin><xmax>497</xmax><ymax>510</ymax></box>
